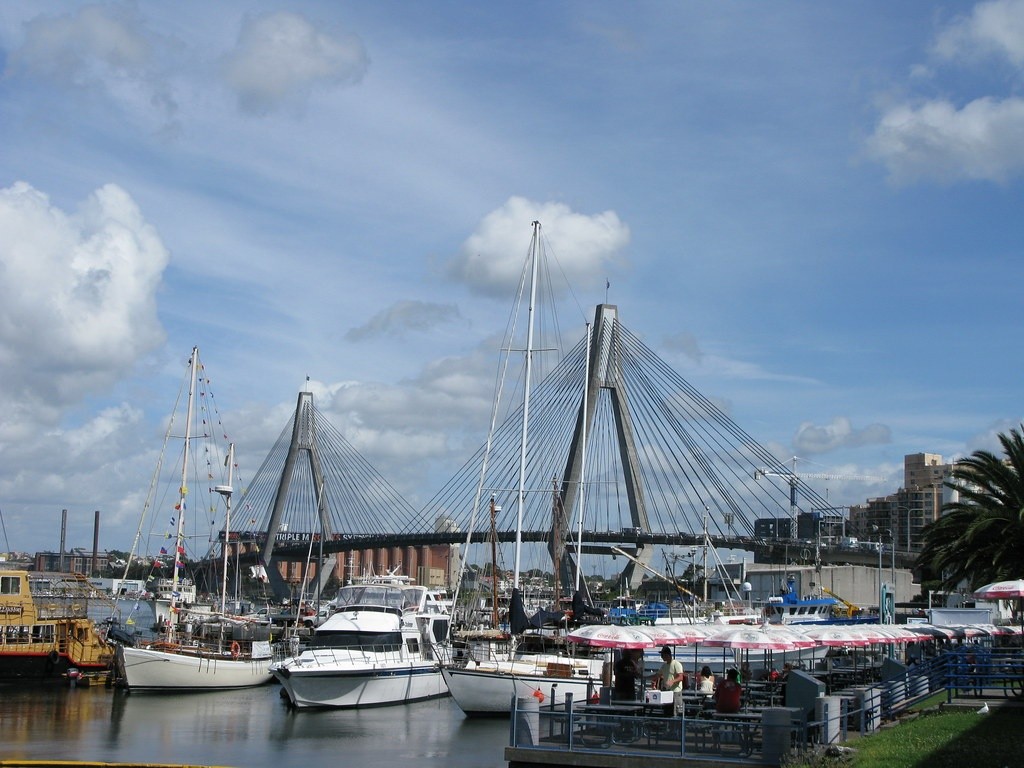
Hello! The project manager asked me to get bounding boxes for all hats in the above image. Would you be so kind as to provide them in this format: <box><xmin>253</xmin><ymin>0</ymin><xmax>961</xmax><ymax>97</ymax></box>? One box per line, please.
<box><xmin>659</xmin><ymin>647</ymin><xmax>671</xmax><ymax>654</ymax></box>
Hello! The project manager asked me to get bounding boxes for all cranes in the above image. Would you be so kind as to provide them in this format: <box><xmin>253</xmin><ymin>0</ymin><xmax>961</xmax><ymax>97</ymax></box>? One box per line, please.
<box><xmin>754</xmin><ymin>455</ymin><xmax>888</xmax><ymax>539</ymax></box>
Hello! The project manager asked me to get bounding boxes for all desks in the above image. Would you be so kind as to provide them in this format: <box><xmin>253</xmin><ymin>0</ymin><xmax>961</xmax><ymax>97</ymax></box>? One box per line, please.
<box><xmin>576</xmin><ymin>663</ymin><xmax>880</xmax><ymax>759</ymax></box>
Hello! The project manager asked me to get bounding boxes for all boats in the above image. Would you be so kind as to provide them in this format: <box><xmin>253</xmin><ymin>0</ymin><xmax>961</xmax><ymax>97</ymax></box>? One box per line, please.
<box><xmin>565</xmin><ymin>618</ymin><xmax>1024</xmax><ymax>699</ymax></box>
<box><xmin>0</xmin><ymin>338</ymin><xmax>518</xmax><ymax>713</ymax></box>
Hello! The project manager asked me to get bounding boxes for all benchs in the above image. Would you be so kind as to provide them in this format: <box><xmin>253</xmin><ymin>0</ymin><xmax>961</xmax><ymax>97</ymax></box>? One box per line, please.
<box><xmin>574</xmin><ymin>721</ymin><xmax>622</xmax><ymax>728</ymax></box>
<box><xmin>709</xmin><ymin>729</ymin><xmax>760</xmax><ymax>735</ymax></box>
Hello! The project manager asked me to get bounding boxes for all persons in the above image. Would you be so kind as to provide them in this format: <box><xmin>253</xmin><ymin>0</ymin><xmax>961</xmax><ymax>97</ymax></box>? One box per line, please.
<box><xmin>611</xmin><ymin>636</ymin><xmax>1019</xmax><ymax>750</ymax></box>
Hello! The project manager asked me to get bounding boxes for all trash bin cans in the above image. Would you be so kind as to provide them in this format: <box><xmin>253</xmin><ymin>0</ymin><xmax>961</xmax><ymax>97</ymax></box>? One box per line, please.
<box><xmin>882</xmin><ymin>656</ymin><xmax>910</xmax><ymax>699</ymax></box>
<box><xmin>786</xmin><ymin>669</ymin><xmax>825</xmax><ymax>744</ymax></box>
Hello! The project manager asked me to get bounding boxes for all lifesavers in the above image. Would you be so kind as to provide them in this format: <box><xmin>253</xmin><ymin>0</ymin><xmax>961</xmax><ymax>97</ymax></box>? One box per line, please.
<box><xmin>49</xmin><ymin>650</ymin><xmax>60</xmax><ymax>665</ymax></box>
<box><xmin>231</xmin><ymin>641</ymin><xmax>240</xmax><ymax>656</ymax></box>
<box><xmin>99</xmin><ymin>630</ymin><xmax>106</xmax><ymax>644</ymax></box>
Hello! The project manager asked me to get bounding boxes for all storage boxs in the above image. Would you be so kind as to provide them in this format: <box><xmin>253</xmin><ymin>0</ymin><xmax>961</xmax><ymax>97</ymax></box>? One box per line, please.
<box><xmin>646</xmin><ymin>690</ymin><xmax>674</xmax><ymax>704</ymax></box>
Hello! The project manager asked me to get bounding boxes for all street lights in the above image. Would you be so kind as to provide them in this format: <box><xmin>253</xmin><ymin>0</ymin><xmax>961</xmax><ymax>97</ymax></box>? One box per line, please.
<box><xmin>872</xmin><ymin>524</ymin><xmax>883</xmax><ymax>624</ymax></box>
<box><xmin>886</xmin><ymin>529</ymin><xmax>896</xmax><ymax>625</ymax></box>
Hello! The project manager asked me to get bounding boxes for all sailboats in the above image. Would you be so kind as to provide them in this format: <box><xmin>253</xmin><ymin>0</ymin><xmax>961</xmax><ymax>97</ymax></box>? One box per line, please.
<box><xmin>433</xmin><ymin>219</ymin><xmax>616</xmax><ymax>722</ymax></box>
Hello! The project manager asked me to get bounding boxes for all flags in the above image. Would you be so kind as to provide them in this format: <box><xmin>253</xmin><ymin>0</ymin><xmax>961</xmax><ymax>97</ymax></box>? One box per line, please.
<box><xmin>123</xmin><ymin>348</ymin><xmax>260</xmax><ymax>628</ymax></box>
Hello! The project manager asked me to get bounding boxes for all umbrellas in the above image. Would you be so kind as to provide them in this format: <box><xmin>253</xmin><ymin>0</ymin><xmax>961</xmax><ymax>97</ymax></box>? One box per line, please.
<box><xmin>563</xmin><ymin>578</ymin><xmax>1024</xmax><ymax>692</ymax></box>
